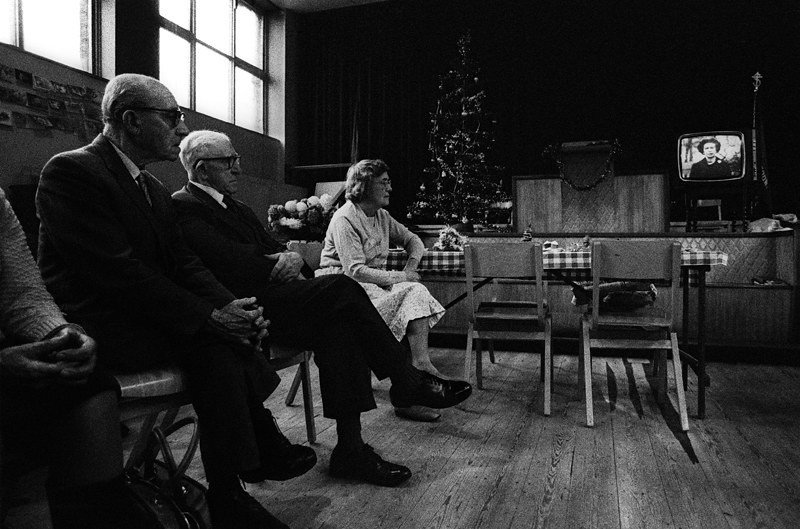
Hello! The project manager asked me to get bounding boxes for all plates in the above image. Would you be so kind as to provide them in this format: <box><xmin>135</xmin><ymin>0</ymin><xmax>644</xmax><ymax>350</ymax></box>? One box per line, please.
<box><xmin>388</xmin><ymin>249</ymin><xmax>404</xmax><ymax>253</ymax></box>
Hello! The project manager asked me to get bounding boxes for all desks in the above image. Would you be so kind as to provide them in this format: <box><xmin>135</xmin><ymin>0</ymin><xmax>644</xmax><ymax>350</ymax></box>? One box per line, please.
<box><xmin>389</xmin><ymin>247</ymin><xmax>729</xmax><ymax>420</ymax></box>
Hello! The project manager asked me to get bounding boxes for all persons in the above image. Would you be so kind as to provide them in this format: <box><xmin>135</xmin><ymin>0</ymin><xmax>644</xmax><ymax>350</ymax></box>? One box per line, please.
<box><xmin>37</xmin><ymin>73</ymin><xmax>316</xmax><ymax>529</ymax></box>
<box><xmin>171</xmin><ymin>130</ymin><xmax>474</xmax><ymax>487</ymax></box>
<box><xmin>316</xmin><ymin>160</ymin><xmax>463</xmax><ymax>420</ymax></box>
<box><xmin>689</xmin><ymin>138</ymin><xmax>731</xmax><ymax>179</ymax></box>
<box><xmin>0</xmin><ymin>182</ymin><xmax>141</xmax><ymax>529</ymax></box>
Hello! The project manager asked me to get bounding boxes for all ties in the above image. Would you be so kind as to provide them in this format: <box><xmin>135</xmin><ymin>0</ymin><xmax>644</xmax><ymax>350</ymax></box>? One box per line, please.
<box><xmin>222</xmin><ymin>195</ymin><xmax>244</xmax><ymax>222</ymax></box>
<box><xmin>137</xmin><ymin>172</ymin><xmax>154</xmax><ymax>208</ymax></box>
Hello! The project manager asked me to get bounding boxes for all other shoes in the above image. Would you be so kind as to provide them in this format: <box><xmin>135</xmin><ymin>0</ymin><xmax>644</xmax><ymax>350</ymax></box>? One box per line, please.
<box><xmin>205</xmin><ymin>478</ymin><xmax>288</xmax><ymax>528</ymax></box>
<box><xmin>232</xmin><ymin>408</ymin><xmax>318</xmax><ymax>481</ymax></box>
<box><xmin>395</xmin><ymin>405</ymin><xmax>442</xmax><ymax>423</ymax></box>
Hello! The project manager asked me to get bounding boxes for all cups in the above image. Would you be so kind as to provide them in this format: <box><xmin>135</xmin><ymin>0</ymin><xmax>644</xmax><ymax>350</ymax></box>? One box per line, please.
<box><xmin>396</xmin><ymin>246</ymin><xmax>404</xmax><ymax>249</ymax></box>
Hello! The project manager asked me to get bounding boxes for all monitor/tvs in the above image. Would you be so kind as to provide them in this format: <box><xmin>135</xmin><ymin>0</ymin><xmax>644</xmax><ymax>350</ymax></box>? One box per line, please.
<box><xmin>677</xmin><ymin>131</ymin><xmax>747</xmax><ymax>183</ymax></box>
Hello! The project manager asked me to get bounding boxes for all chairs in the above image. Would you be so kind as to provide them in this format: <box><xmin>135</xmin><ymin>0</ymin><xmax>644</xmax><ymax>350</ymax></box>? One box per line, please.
<box><xmin>462</xmin><ymin>242</ymin><xmax>554</xmax><ymax>416</ymax></box>
<box><xmin>577</xmin><ymin>242</ymin><xmax>689</xmax><ymax>432</ymax></box>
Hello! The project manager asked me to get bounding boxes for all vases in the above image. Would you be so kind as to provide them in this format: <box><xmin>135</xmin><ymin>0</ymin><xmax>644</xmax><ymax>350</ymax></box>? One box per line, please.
<box><xmin>286</xmin><ymin>243</ymin><xmax>323</xmax><ymax>270</ymax></box>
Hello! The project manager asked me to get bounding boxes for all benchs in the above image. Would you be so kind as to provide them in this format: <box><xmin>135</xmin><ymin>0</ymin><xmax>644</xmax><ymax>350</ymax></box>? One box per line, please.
<box><xmin>115</xmin><ymin>243</ymin><xmax>317</xmax><ymax>470</ymax></box>
<box><xmin>388</xmin><ymin>230</ymin><xmax>800</xmax><ymax>364</ymax></box>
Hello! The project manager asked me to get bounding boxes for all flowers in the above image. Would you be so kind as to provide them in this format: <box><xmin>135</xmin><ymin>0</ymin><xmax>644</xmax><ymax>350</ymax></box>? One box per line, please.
<box><xmin>266</xmin><ymin>196</ymin><xmax>337</xmax><ymax>244</ymax></box>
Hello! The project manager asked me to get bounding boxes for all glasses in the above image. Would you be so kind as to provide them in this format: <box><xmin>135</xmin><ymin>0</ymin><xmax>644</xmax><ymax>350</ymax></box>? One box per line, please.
<box><xmin>373</xmin><ymin>180</ymin><xmax>392</xmax><ymax>187</ymax></box>
<box><xmin>132</xmin><ymin>105</ymin><xmax>186</xmax><ymax>127</ymax></box>
<box><xmin>193</xmin><ymin>154</ymin><xmax>242</xmax><ymax>170</ymax></box>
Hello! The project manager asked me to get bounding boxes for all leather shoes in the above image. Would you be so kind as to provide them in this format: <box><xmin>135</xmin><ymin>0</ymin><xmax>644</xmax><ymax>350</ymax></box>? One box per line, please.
<box><xmin>388</xmin><ymin>369</ymin><xmax>472</xmax><ymax>409</ymax></box>
<box><xmin>327</xmin><ymin>442</ymin><xmax>414</xmax><ymax>488</ymax></box>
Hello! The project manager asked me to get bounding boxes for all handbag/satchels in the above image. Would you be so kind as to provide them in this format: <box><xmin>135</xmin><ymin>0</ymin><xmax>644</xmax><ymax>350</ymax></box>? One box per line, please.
<box><xmin>124</xmin><ymin>419</ymin><xmax>215</xmax><ymax>529</ymax></box>
